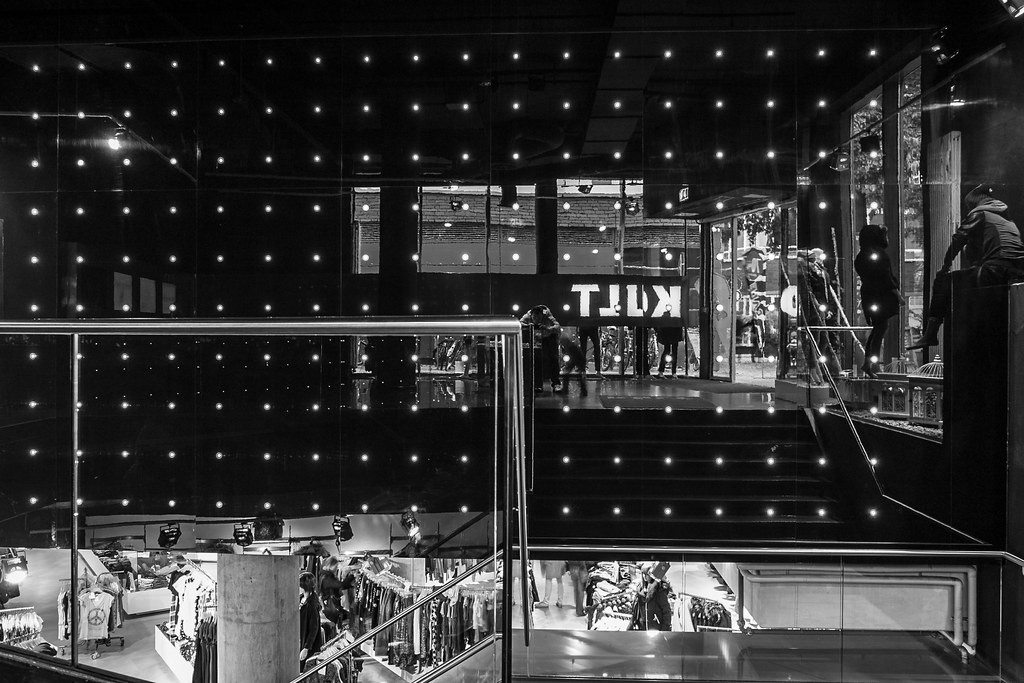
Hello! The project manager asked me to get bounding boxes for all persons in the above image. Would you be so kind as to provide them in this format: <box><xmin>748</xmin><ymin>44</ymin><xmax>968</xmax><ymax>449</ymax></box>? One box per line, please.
<box><xmin>559</xmin><ymin>332</ymin><xmax>590</xmax><ymax>398</ymax></box>
<box><xmin>574</xmin><ymin>325</ymin><xmax>606</xmax><ymax>381</ymax></box>
<box><xmin>852</xmin><ymin>224</ymin><xmax>906</xmax><ymax>380</ymax></box>
<box><xmin>569</xmin><ymin>560</ymin><xmax>596</xmax><ymax>617</ymax></box>
<box><xmin>319</xmin><ymin>556</ymin><xmax>358</xmax><ymax>635</ymax></box>
<box><xmin>298</xmin><ymin>572</ymin><xmax>323</xmax><ymax>676</ymax></box>
<box><xmin>903</xmin><ymin>183</ymin><xmax>1024</xmax><ymax>352</ymax></box>
<box><xmin>637</xmin><ymin>560</ymin><xmax>673</xmax><ymax>631</ymax></box>
<box><xmin>794</xmin><ymin>245</ymin><xmax>834</xmax><ymax>385</ymax></box>
<box><xmin>655</xmin><ymin>327</ymin><xmax>684</xmax><ymax>380</ymax></box>
<box><xmin>534</xmin><ymin>558</ymin><xmax>569</xmax><ymax>609</ymax></box>
<box><xmin>520</xmin><ymin>304</ymin><xmax>564</xmax><ymax>394</ymax></box>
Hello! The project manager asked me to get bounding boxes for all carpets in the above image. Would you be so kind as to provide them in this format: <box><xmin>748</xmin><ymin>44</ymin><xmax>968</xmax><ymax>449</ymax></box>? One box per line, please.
<box><xmin>651</xmin><ymin>377</ymin><xmax>776</xmax><ymax>394</ymax></box>
<box><xmin>599</xmin><ymin>394</ymin><xmax>720</xmax><ymax>410</ymax></box>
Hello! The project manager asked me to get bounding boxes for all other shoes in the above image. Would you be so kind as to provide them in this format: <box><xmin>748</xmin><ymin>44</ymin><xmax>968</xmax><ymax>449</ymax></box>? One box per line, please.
<box><xmin>535</xmin><ymin>384</ymin><xmax>587</xmax><ymax>396</ymax></box>
<box><xmin>861</xmin><ymin>364</ymin><xmax>879</xmax><ymax>380</ymax></box>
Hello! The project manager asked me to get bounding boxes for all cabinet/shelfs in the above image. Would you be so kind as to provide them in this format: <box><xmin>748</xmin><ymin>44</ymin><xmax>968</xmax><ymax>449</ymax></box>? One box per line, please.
<box><xmin>154</xmin><ymin>557</ymin><xmax>217</xmax><ymax>683</ymax></box>
<box><xmin>906</xmin><ymin>353</ymin><xmax>944</xmax><ymax>429</ymax></box>
<box><xmin>0</xmin><ymin>606</ymin><xmax>44</xmax><ymax>646</ymax></box>
<box><xmin>678</xmin><ymin>592</ymin><xmax>732</xmax><ymax>633</ymax></box>
<box><xmin>59</xmin><ymin>567</ymin><xmax>127</xmax><ymax>661</ymax></box>
<box><xmin>876</xmin><ymin>353</ymin><xmax>918</xmax><ymax>418</ymax></box>
<box><xmin>834</xmin><ymin>367</ymin><xmax>877</xmax><ymax>408</ymax></box>
<box><xmin>351</xmin><ymin>561</ymin><xmax>504</xmax><ymax>674</ymax></box>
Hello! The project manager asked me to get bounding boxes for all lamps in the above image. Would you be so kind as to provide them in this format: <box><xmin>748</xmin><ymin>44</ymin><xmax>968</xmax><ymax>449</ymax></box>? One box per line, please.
<box><xmin>233</xmin><ymin>520</ymin><xmax>253</xmax><ymax>548</ymax></box>
<box><xmin>157</xmin><ymin>522</ymin><xmax>183</xmax><ymax>549</ymax></box>
<box><xmin>0</xmin><ymin>548</ymin><xmax>29</xmax><ymax>584</ymax></box>
<box><xmin>401</xmin><ymin>511</ymin><xmax>422</xmax><ymax>545</ymax></box>
<box><xmin>332</xmin><ymin>513</ymin><xmax>354</xmax><ymax>542</ymax></box>
